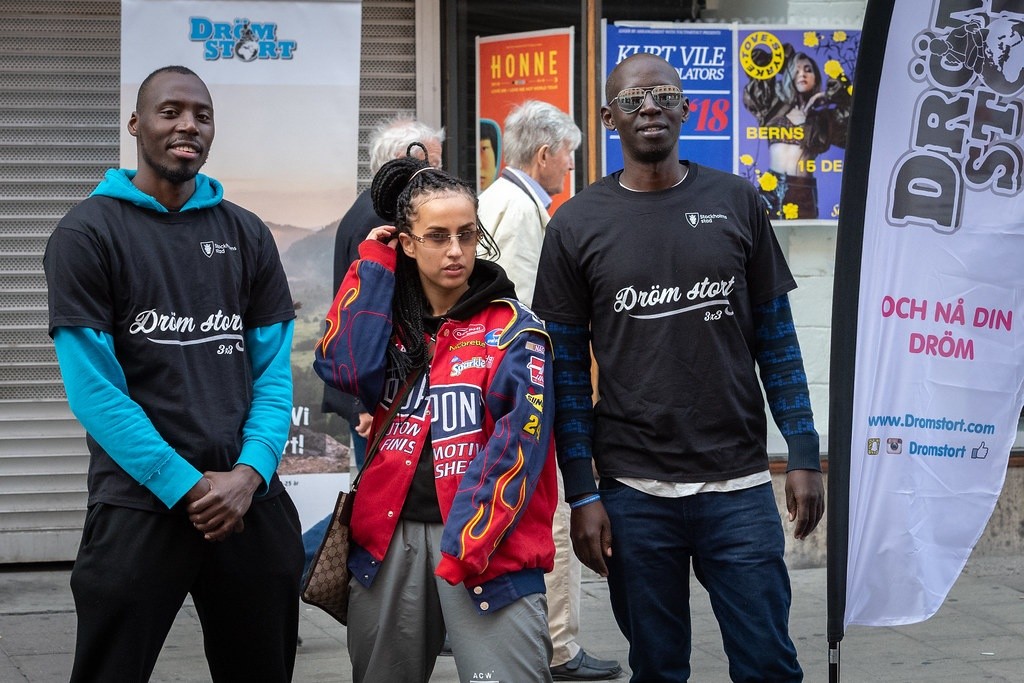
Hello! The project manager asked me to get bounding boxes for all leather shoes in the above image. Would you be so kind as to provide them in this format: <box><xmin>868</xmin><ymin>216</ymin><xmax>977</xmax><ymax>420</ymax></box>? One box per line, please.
<box><xmin>550</xmin><ymin>648</ymin><xmax>622</xmax><ymax>681</ymax></box>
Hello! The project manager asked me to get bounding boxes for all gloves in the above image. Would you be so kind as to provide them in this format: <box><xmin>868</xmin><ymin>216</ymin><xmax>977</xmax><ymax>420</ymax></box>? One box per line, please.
<box><xmin>770</xmin><ymin>43</ymin><xmax>794</xmax><ymax>74</ymax></box>
<box><xmin>826</xmin><ymin>72</ymin><xmax>853</xmax><ymax>103</ymax></box>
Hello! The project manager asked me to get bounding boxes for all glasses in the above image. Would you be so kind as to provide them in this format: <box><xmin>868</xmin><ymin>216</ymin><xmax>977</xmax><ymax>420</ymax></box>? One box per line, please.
<box><xmin>409</xmin><ymin>228</ymin><xmax>483</xmax><ymax>248</ymax></box>
<box><xmin>609</xmin><ymin>85</ymin><xmax>684</xmax><ymax>114</ymax></box>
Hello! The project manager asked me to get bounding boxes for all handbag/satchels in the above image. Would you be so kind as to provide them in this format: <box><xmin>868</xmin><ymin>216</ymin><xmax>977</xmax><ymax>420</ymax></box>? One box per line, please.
<box><xmin>301</xmin><ymin>491</ymin><xmax>352</xmax><ymax>625</ymax></box>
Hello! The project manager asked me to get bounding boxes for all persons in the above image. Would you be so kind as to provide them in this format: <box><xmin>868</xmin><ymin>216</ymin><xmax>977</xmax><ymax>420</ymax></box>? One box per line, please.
<box><xmin>744</xmin><ymin>49</ymin><xmax>854</xmax><ymax>220</ymax></box>
<box><xmin>313</xmin><ymin>53</ymin><xmax>825</xmax><ymax>683</ymax></box>
<box><xmin>43</xmin><ymin>66</ymin><xmax>304</xmax><ymax>683</ymax></box>
<box><xmin>479</xmin><ymin>122</ymin><xmax>498</xmax><ymax>192</ymax></box>
